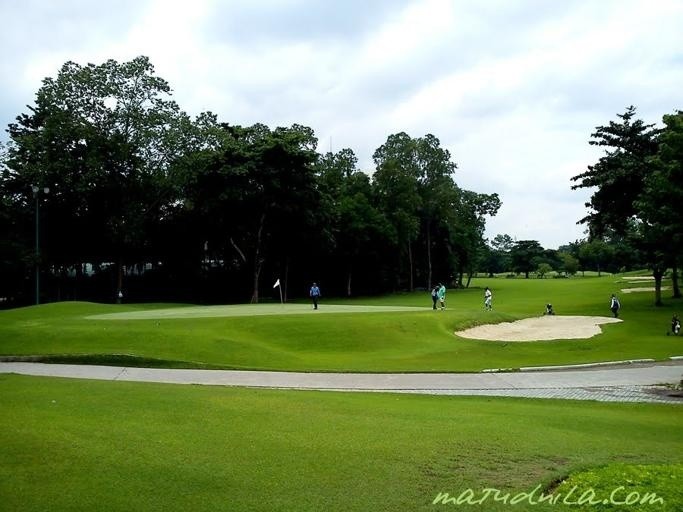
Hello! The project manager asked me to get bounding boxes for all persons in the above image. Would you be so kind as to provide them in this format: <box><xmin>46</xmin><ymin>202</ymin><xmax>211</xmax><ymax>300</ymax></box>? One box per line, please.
<box><xmin>308</xmin><ymin>281</ymin><xmax>321</xmax><ymax>310</ymax></box>
<box><xmin>544</xmin><ymin>303</ymin><xmax>555</xmax><ymax>315</ymax></box>
<box><xmin>609</xmin><ymin>293</ymin><xmax>621</xmax><ymax>317</ymax></box>
<box><xmin>484</xmin><ymin>286</ymin><xmax>492</xmax><ymax>312</ymax></box>
<box><xmin>436</xmin><ymin>281</ymin><xmax>446</xmax><ymax>312</ymax></box>
<box><xmin>431</xmin><ymin>286</ymin><xmax>439</xmax><ymax>310</ymax></box>
<box><xmin>671</xmin><ymin>312</ymin><xmax>680</xmax><ymax>335</ymax></box>
